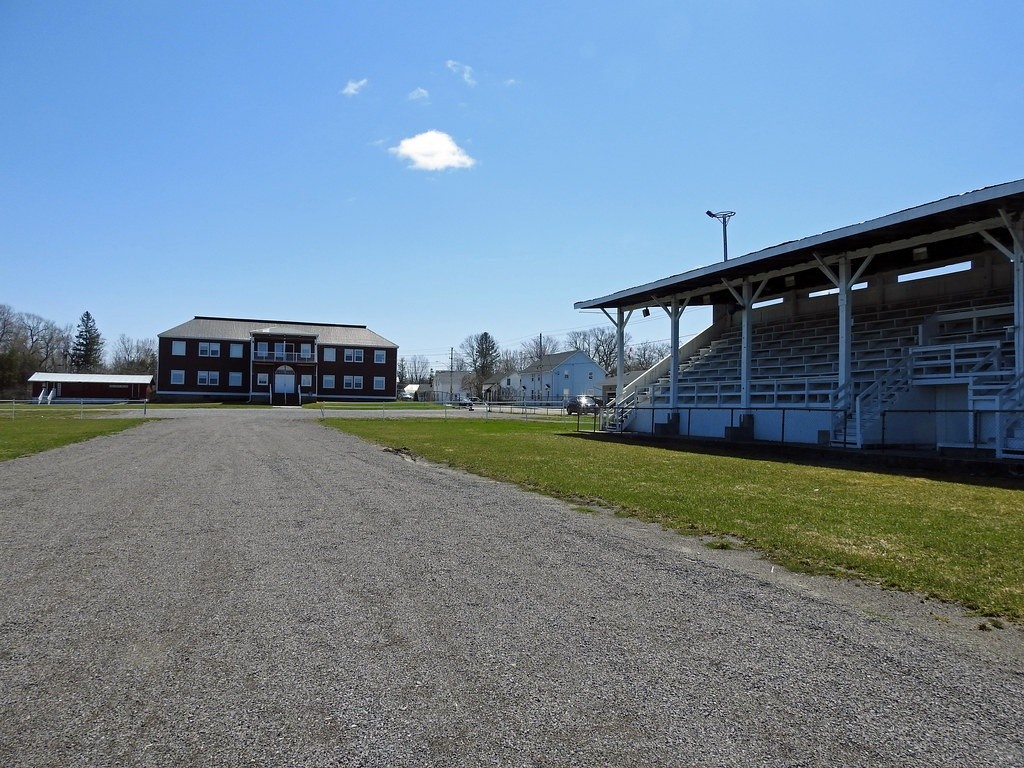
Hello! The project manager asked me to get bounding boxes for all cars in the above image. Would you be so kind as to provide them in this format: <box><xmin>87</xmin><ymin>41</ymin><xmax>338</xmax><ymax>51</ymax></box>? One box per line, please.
<box><xmin>453</xmin><ymin>397</ymin><xmax>474</xmax><ymax>406</ymax></box>
<box><xmin>566</xmin><ymin>395</ymin><xmax>600</xmax><ymax>416</ymax></box>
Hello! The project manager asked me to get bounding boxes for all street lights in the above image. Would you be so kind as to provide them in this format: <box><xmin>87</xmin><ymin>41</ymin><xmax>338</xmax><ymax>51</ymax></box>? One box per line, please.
<box><xmin>705</xmin><ymin>210</ymin><xmax>736</xmax><ymax>262</ymax></box>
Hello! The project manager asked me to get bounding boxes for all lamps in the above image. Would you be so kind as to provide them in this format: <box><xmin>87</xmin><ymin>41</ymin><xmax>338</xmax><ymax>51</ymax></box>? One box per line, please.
<box><xmin>642</xmin><ymin>306</ymin><xmax>650</xmax><ymax>318</ymax></box>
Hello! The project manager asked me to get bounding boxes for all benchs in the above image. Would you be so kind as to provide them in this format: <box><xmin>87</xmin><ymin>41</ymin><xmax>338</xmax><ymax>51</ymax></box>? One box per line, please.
<box><xmin>632</xmin><ymin>287</ymin><xmax>1018</xmax><ymax>403</ymax></box>
